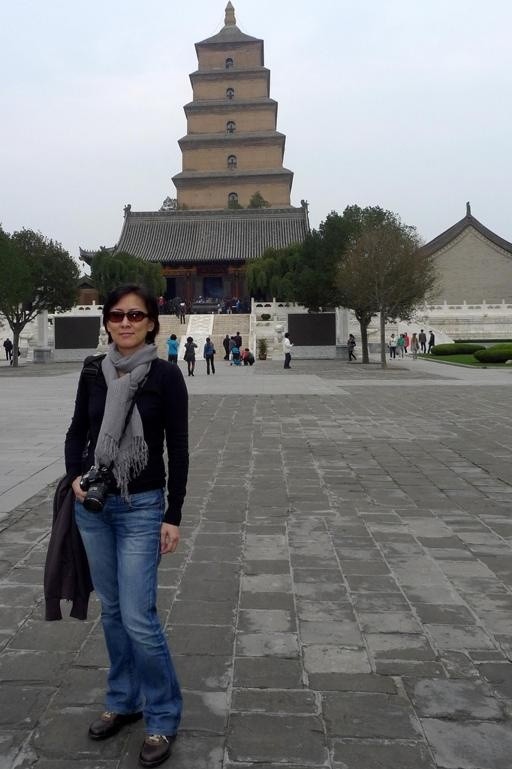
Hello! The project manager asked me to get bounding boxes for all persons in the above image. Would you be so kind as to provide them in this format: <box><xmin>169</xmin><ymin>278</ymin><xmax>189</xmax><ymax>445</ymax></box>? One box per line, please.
<box><xmin>282</xmin><ymin>333</ymin><xmax>294</xmax><ymax>368</ymax></box>
<box><xmin>428</xmin><ymin>331</ymin><xmax>434</xmax><ymax>353</ymax></box>
<box><xmin>347</xmin><ymin>334</ymin><xmax>357</xmax><ymax>361</ymax></box>
<box><xmin>3</xmin><ymin>338</ymin><xmax>12</xmax><ymax>360</ymax></box>
<box><xmin>159</xmin><ymin>295</ymin><xmax>255</xmax><ymax>376</ymax></box>
<box><xmin>64</xmin><ymin>286</ymin><xmax>189</xmax><ymax>768</ymax></box>
<box><xmin>410</xmin><ymin>333</ymin><xmax>419</xmax><ymax>359</ymax></box>
<box><xmin>388</xmin><ymin>332</ymin><xmax>410</xmax><ymax>360</ymax></box>
<box><xmin>419</xmin><ymin>329</ymin><xmax>426</xmax><ymax>353</ymax></box>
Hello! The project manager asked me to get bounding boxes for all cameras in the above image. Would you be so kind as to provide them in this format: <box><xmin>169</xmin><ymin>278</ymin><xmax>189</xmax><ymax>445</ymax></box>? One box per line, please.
<box><xmin>80</xmin><ymin>466</ymin><xmax>121</xmax><ymax>514</ymax></box>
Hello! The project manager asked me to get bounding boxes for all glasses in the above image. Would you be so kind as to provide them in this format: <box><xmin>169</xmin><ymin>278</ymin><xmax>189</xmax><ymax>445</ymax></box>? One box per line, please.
<box><xmin>104</xmin><ymin>310</ymin><xmax>148</xmax><ymax>323</ymax></box>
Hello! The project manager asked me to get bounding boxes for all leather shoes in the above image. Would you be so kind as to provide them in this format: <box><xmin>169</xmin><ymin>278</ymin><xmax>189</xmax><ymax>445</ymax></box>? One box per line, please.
<box><xmin>137</xmin><ymin>730</ymin><xmax>177</xmax><ymax>768</ymax></box>
<box><xmin>87</xmin><ymin>708</ymin><xmax>145</xmax><ymax>742</ymax></box>
